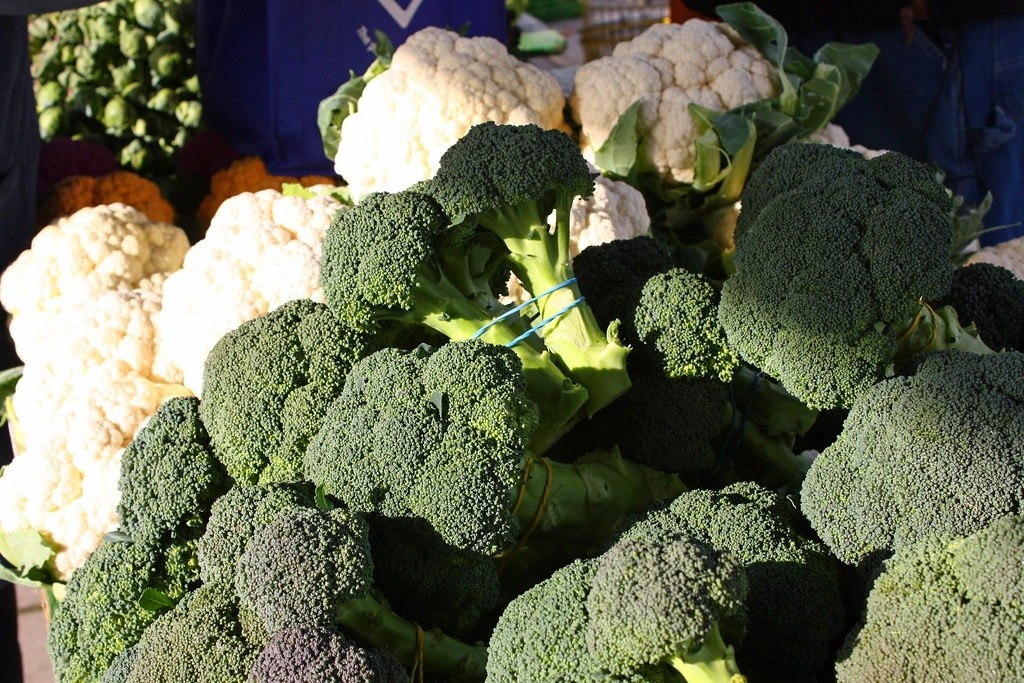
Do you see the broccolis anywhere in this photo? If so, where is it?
[0,2,1024,683]
